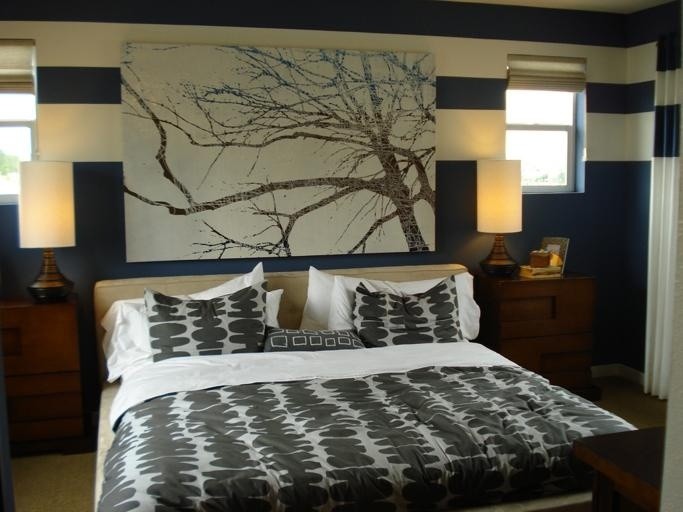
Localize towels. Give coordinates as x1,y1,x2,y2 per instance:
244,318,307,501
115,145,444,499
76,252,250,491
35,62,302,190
518,264,561,280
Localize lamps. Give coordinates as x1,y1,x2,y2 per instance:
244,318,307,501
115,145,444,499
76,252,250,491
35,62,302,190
475,157,522,277
18,158,78,302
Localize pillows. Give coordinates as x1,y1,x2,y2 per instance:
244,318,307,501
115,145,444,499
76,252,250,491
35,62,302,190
350,274,466,349
141,278,268,364
292,264,333,332
100,260,265,383
265,288,286,330
260,321,366,353
326,271,481,343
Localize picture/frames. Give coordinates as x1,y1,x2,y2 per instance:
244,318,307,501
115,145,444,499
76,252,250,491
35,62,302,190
537,236,569,276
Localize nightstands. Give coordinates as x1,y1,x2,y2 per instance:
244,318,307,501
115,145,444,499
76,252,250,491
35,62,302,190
2,296,99,456
471,266,598,394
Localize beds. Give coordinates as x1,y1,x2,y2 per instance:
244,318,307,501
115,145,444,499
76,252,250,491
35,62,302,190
86,251,638,512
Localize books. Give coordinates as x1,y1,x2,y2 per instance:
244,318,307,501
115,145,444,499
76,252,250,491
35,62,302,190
519,274,562,279
521,265,561,274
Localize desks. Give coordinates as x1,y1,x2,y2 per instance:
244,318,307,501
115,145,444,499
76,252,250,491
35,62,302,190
564,424,664,512
